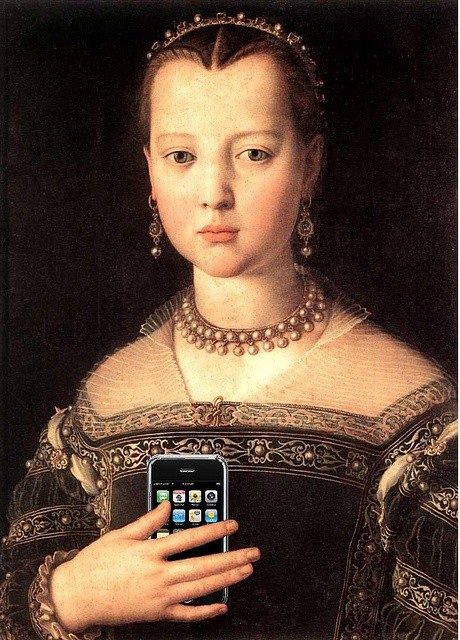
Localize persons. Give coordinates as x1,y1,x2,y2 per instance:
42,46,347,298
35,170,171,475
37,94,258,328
2,11,458,639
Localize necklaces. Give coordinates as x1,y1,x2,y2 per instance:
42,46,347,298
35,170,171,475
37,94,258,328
171,268,328,357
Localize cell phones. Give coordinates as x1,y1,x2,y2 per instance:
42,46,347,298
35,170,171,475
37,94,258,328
143,454,229,609
